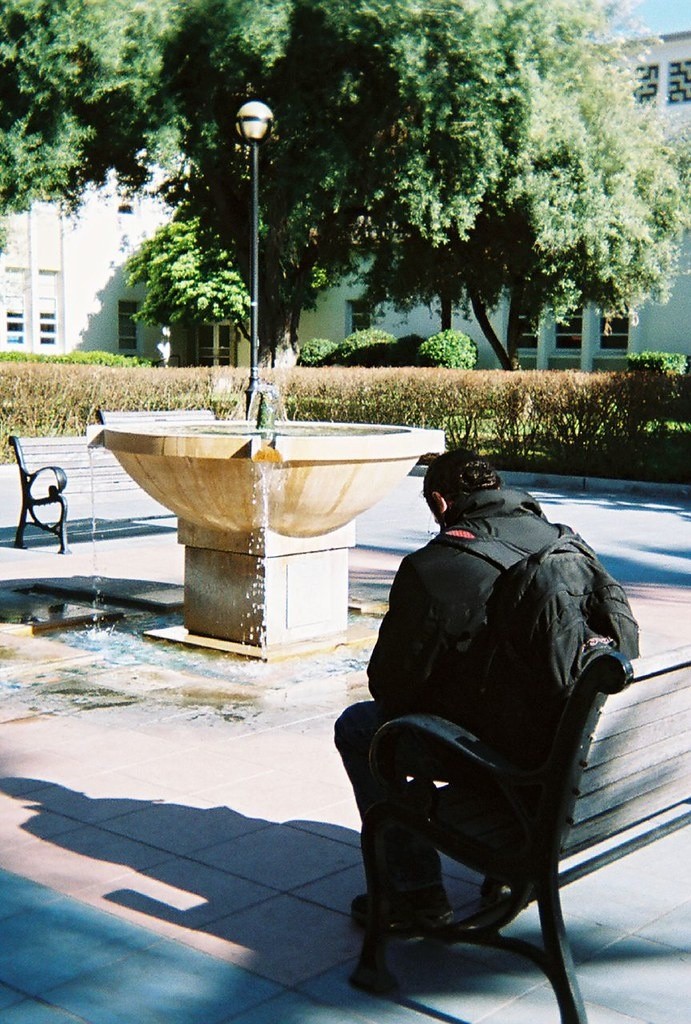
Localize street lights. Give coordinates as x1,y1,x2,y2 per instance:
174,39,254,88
237,100,275,419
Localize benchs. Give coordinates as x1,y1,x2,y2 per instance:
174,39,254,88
96,409,215,424
8,436,154,554
347,648,691,1024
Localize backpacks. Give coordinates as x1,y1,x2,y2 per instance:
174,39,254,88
429,524,640,764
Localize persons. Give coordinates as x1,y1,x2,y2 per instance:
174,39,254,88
331,447,596,933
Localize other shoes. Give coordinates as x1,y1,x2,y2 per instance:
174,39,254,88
350,886,453,932
